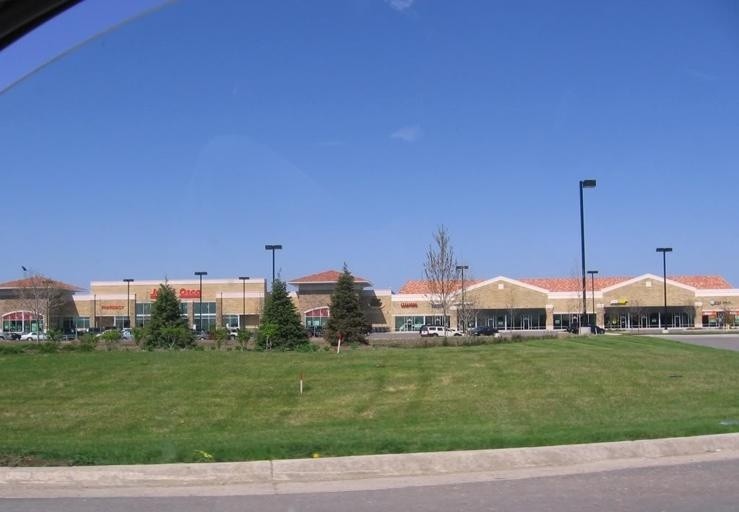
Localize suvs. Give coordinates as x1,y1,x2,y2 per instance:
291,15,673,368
565,322,606,335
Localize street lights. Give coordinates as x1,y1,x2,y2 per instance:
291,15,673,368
456,264,469,333
238,276,250,332
577,176,596,336
264,243,283,287
587,270,599,328
193,270,207,330
122,278,135,330
655,247,674,334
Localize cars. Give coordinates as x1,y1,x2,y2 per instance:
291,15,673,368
190,325,240,342
0,325,136,342
305,325,325,339
419,323,500,338
